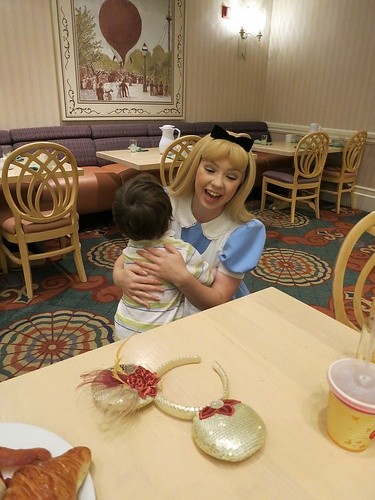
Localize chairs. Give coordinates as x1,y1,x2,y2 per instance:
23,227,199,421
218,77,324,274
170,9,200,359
160,135,201,186
0,141,86,297
333,210,375,331
320,130,368,214
260,131,329,222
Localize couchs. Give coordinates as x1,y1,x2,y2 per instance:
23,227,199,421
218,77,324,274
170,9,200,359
0,120,295,220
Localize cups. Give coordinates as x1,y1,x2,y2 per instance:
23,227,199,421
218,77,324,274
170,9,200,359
326,359,375,452
261,135,267,144
357,320,375,364
129,140,137,152
286,135,291,142
2,146,11,158
0,158,7,170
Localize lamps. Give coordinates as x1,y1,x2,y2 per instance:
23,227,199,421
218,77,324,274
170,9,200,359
240,26,264,43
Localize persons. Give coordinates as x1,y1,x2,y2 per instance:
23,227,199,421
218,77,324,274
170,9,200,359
112,124,267,310
113,172,214,340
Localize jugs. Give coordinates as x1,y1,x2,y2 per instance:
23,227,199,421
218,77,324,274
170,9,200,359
159,125,181,154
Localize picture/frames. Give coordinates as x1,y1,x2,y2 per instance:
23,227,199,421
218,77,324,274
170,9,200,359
50,0,187,121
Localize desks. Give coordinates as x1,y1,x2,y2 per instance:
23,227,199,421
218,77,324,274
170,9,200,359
0,286,375,500
95,144,258,171
253,141,342,156
0,155,84,184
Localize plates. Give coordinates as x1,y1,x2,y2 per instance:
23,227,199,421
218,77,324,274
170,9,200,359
0,423,96,500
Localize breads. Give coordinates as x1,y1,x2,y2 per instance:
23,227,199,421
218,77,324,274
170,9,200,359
0,445,92,500
